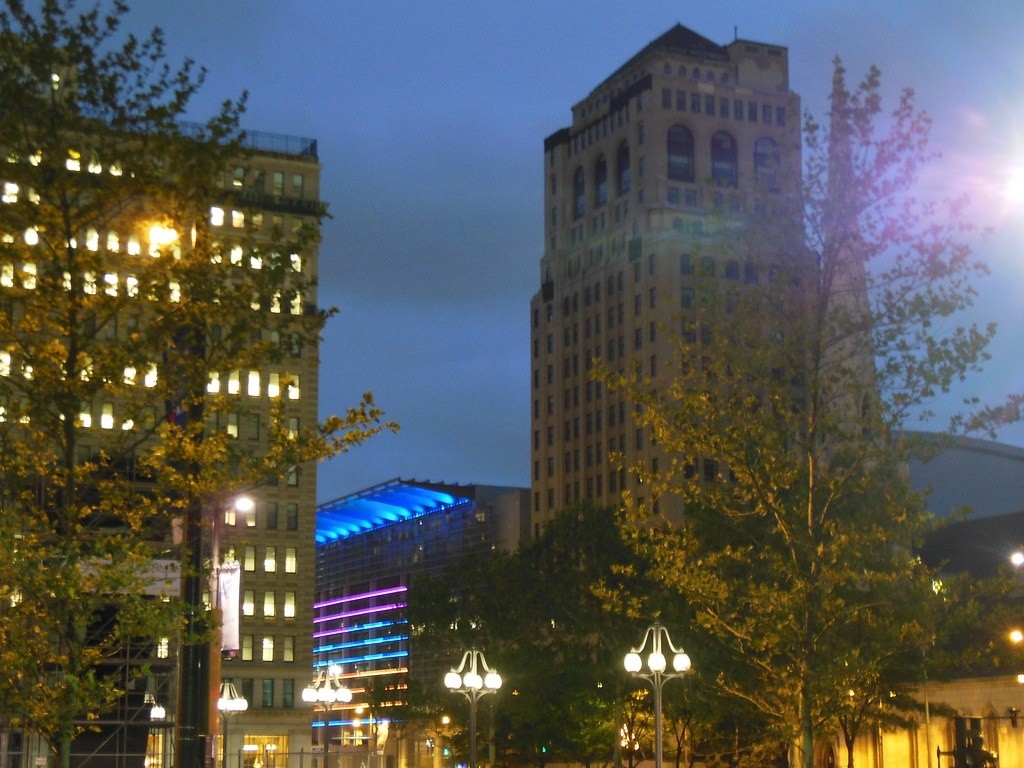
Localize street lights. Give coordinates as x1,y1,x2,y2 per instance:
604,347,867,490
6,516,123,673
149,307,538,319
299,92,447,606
623,606,694,768
219,679,248,768
301,667,352,768
444,641,503,768
151,702,165,768
206,496,255,768
